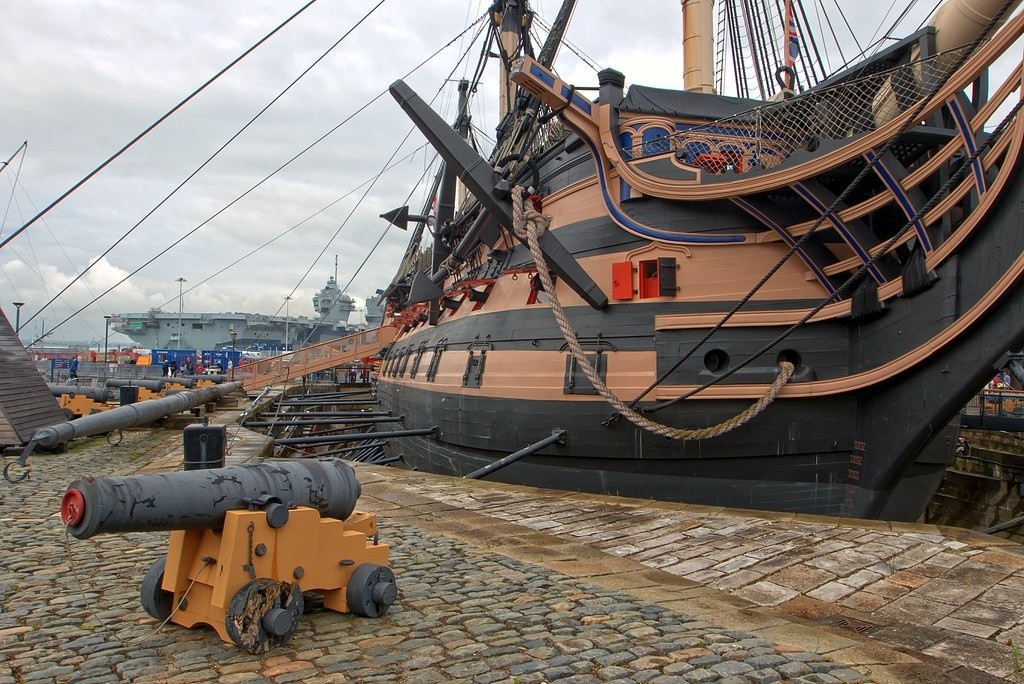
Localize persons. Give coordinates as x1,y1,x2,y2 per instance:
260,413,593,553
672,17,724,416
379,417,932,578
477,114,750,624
992,372,1010,390
68,353,80,385
361,369,366,383
227,358,232,369
161,357,223,378
350,364,357,383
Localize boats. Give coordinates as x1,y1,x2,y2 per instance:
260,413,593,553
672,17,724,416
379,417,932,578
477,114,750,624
110,255,387,357
368,0,1023,533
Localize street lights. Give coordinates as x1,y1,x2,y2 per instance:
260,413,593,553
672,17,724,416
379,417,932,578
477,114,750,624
229,329,237,381
284,296,294,349
102,314,111,379
176,277,186,348
12,302,25,336
